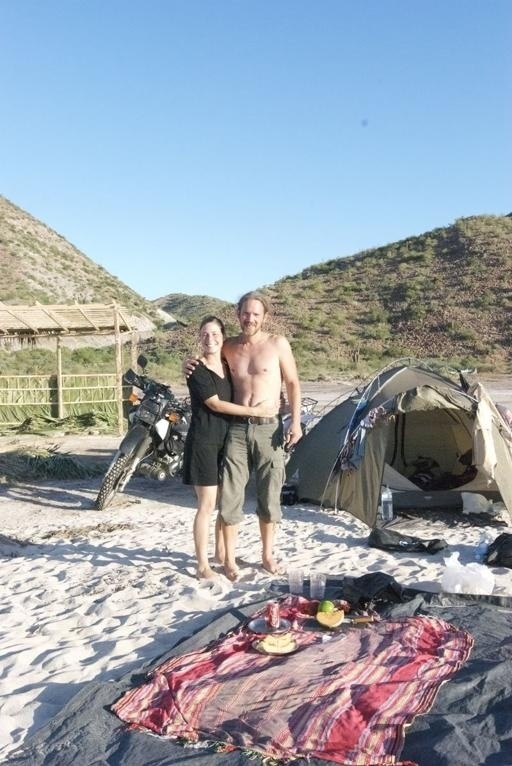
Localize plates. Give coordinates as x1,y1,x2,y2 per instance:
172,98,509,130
247,618,292,635
250,639,300,656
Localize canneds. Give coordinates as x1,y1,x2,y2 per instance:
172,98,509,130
266,601,280,629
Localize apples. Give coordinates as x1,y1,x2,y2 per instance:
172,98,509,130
318,601,334,612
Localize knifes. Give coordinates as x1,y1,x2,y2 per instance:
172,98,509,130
342,614,375,625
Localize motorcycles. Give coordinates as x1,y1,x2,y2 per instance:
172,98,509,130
95,349,318,511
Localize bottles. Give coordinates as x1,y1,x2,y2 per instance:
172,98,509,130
380,484,394,522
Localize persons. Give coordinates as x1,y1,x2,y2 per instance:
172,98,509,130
181,290,303,583
181,316,283,582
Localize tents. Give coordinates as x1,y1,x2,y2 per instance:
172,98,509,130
283,357,512,535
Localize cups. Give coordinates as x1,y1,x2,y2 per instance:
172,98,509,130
287,569,304,594
310,573,326,600
